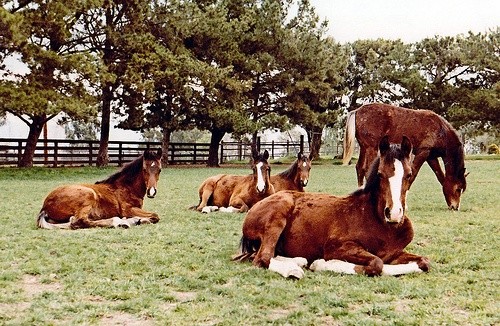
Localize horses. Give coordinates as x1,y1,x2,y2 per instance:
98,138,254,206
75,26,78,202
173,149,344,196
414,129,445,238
37,147,163,229
188,150,275,213
342,103,470,211
270,148,314,193
232,134,432,280
